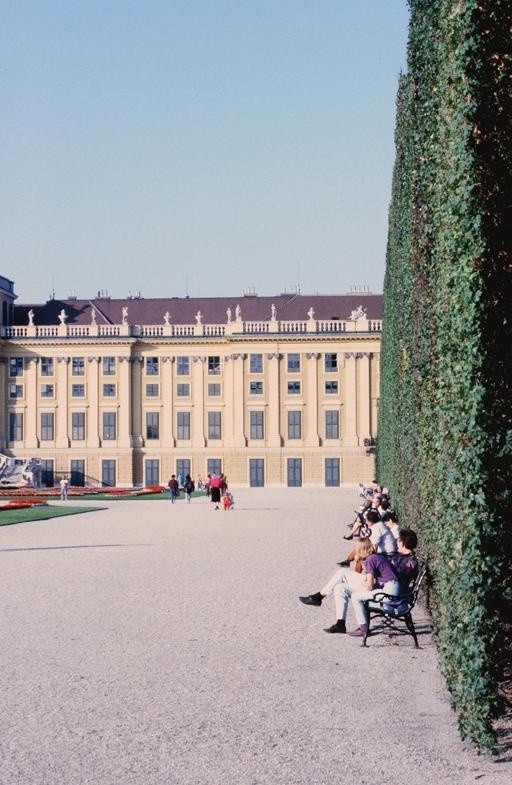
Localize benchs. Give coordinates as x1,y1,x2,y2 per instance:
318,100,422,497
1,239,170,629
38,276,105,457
360,556,428,650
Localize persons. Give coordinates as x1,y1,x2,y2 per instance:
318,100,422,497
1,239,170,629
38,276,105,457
333,477,403,570
58,474,70,501
298,527,420,634
346,537,403,637
166,470,233,513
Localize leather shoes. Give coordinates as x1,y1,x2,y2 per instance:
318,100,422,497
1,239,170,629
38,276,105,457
343,534,359,540
338,560,349,566
299,596,321,605
323,624,346,633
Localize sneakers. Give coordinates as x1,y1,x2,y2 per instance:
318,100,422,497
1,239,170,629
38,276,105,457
348,628,367,636
